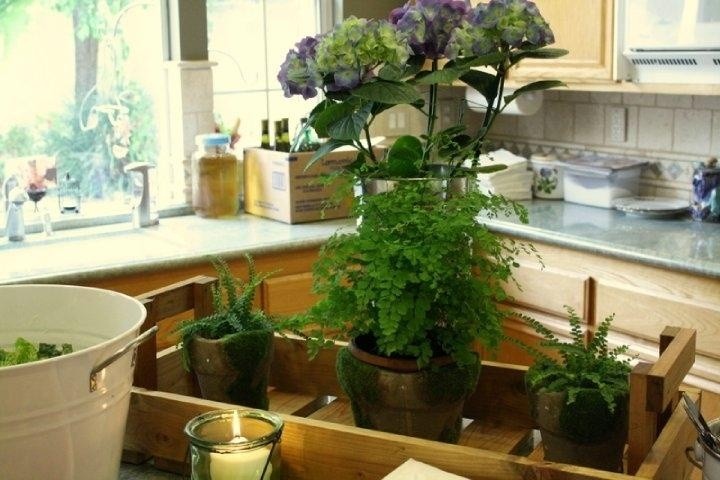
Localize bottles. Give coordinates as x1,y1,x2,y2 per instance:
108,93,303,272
260,118,309,152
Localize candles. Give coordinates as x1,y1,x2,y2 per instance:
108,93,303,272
208,411,272,480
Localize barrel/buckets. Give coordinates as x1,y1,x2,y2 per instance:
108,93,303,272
1,284,158,479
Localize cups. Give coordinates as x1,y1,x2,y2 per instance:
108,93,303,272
184,407,284,480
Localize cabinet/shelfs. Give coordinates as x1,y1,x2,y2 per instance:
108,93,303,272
419,0,616,85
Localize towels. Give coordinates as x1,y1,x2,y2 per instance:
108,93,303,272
468,148,534,201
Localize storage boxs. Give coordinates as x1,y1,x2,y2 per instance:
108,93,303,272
555,158,650,210
243,146,356,224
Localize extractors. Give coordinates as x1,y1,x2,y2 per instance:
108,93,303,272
618,44,720,86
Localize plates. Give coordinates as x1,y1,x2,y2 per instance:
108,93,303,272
610,198,690,217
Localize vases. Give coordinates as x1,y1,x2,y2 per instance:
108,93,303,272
353,165,469,237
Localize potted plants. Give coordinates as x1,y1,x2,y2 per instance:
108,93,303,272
272,131,546,445
156,252,314,406
487,305,641,473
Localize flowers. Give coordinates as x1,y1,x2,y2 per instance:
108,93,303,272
276,1,569,181
